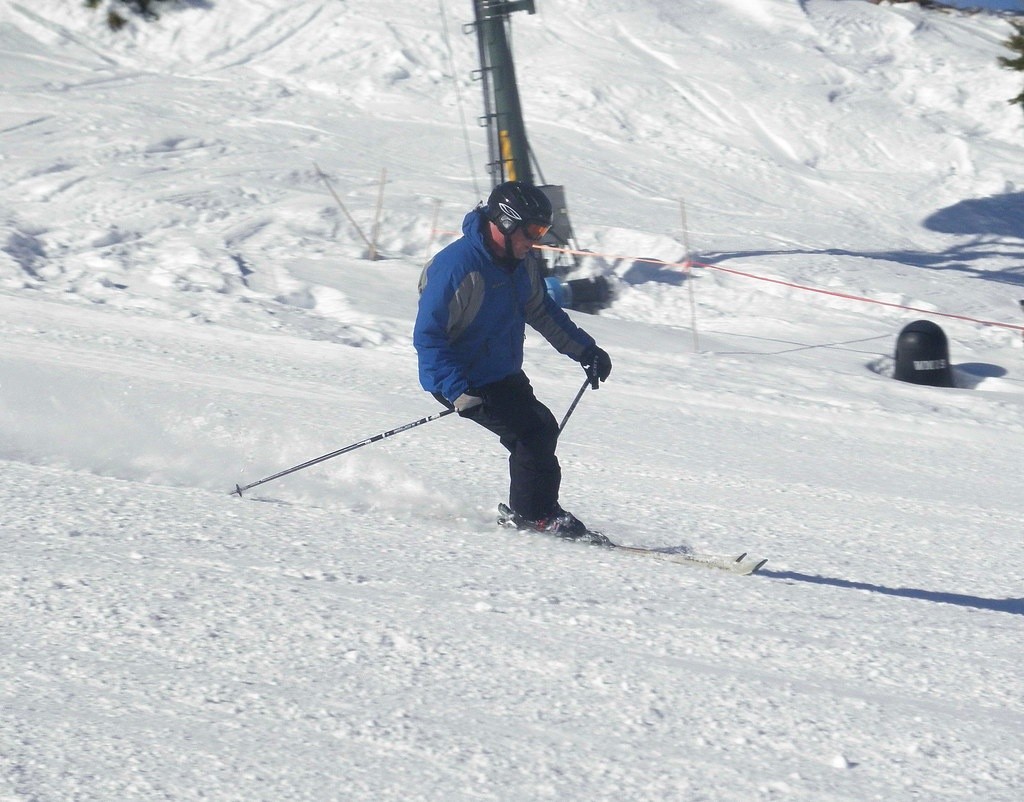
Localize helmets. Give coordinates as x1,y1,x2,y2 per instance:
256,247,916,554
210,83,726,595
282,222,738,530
487,181,552,237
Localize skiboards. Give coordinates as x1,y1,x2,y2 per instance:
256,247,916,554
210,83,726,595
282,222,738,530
499,499,768,578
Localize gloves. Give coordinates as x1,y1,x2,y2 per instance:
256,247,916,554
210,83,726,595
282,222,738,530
453,387,489,418
581,344,612,390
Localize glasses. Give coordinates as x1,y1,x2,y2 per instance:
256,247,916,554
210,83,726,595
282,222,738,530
518,218,552,242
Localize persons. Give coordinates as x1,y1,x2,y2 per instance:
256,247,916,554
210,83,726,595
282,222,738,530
413,182,614,538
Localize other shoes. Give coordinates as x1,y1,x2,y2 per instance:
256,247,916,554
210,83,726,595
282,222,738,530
511,506,587,542
